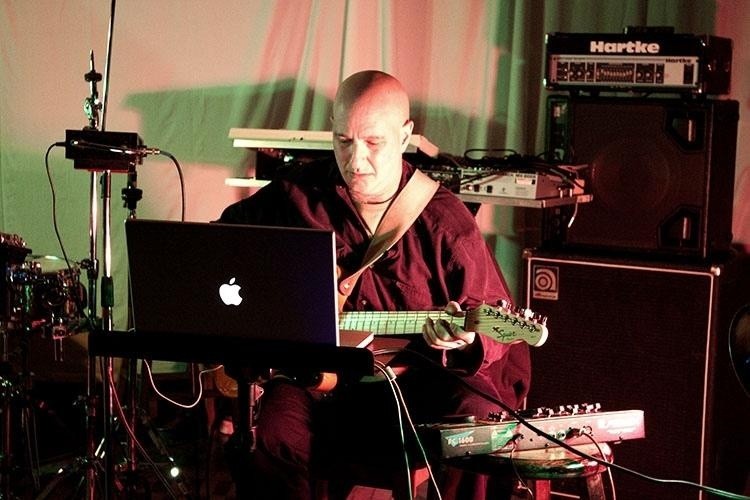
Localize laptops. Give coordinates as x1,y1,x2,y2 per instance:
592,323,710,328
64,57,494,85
124,219,374,348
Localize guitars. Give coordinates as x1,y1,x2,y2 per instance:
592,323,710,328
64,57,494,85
339,299,550,348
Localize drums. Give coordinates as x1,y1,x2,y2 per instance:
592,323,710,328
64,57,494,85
9,254,84,340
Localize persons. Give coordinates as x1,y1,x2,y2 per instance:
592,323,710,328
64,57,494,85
206,70,533,500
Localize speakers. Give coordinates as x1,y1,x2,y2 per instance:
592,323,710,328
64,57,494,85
518,245,750,500
545,93,741,264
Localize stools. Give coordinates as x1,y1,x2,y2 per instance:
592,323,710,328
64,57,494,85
455,442,614,500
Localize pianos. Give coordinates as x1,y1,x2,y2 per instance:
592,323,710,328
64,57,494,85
254,146,586,201
